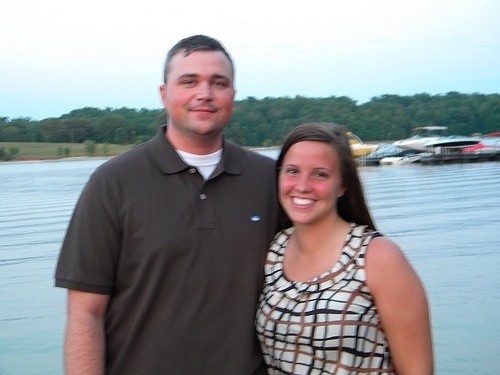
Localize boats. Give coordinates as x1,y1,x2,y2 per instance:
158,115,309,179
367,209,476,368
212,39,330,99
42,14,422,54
395,125,481,151
345,132,379,157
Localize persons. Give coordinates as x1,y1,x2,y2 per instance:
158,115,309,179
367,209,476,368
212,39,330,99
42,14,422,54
54,33,277,374
254,122,435,374
